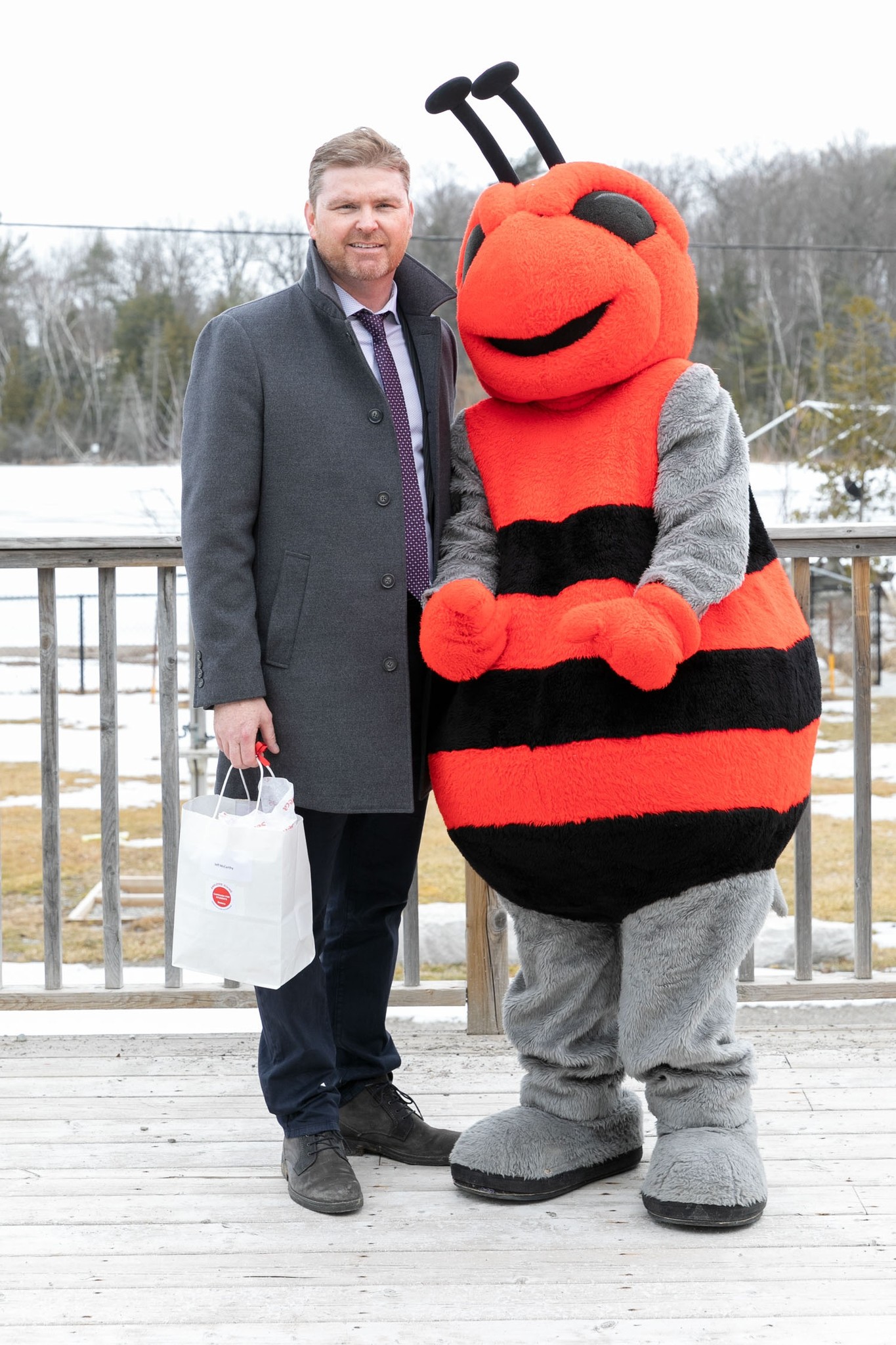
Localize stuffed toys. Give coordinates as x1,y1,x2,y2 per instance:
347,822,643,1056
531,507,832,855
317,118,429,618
423,62,820,1233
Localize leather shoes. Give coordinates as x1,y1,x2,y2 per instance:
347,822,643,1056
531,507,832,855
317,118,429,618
280,1127,364,1213
337,1083,462,1167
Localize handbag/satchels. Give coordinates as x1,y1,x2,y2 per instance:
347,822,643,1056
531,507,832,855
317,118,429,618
173,752,316,990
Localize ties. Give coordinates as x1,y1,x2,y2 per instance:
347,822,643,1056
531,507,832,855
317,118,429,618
351,308,430,603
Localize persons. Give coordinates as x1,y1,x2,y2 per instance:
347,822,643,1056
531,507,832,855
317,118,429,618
182,128,459,1213
